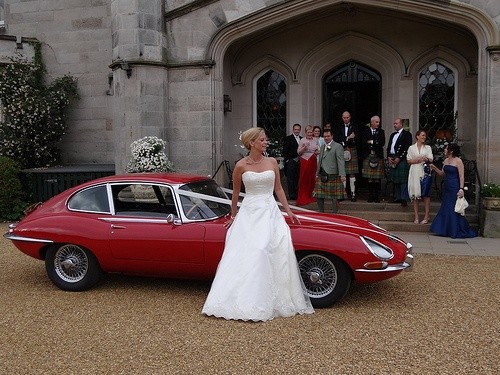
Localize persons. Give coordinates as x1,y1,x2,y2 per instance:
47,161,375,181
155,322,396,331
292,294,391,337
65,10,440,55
201,127,316,322
386,118,412,207
406,129,435,224
430,144,477,239
296,125,320,206
314,129,347,214
337,111,359,202
312,123,331,162
282,124,303,201
360,116,386,203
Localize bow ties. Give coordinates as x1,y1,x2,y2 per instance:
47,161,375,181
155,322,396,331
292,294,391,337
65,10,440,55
394,132,399,134
346,125,349,127
324,145,331,152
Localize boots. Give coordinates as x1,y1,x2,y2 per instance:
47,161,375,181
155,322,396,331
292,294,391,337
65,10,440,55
350,177,357,202
375,181,381,203
367,182,374,202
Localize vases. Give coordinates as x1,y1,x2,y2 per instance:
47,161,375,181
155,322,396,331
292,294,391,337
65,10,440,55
129,184,168,202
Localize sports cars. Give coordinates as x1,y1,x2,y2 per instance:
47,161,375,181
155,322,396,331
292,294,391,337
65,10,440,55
2,172,414,309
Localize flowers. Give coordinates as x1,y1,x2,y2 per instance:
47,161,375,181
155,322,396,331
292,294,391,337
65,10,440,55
124,136,175,174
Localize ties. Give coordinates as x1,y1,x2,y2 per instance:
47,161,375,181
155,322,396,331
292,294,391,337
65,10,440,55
373,131,375,137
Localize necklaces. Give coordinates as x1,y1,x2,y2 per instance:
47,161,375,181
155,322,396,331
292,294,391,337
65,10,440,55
249,156,264,165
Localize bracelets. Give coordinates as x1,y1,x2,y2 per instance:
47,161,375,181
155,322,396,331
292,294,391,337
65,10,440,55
231,216,234,220
459,187,464,190
287,210,292,215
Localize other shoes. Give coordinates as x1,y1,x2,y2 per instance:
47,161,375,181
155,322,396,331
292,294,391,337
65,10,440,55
401,199,407,206
414,216,419,224
390,199,402,203
420,216,430,224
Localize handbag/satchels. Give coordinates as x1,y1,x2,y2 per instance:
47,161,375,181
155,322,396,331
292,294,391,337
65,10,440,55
455,196,469,216
321,172,328,182
343,151,351,161
369,157,379,168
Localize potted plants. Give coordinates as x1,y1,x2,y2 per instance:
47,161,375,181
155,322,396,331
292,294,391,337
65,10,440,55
480,183,500,210
